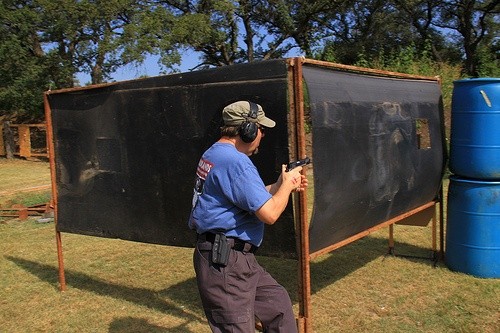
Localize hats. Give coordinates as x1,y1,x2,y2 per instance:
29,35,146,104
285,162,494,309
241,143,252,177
221,100,276,127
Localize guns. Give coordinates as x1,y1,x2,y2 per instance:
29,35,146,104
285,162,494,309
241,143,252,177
288,156,310,172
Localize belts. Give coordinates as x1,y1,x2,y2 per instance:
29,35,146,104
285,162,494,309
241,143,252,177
198,231,257,253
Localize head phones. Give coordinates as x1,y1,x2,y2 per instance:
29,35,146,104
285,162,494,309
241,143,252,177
239,99,258,144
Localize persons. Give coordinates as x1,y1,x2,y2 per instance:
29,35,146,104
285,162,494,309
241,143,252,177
188,100,309,333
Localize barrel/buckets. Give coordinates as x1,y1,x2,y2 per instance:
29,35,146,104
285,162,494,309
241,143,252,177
445,179,500,279
448,78,500,179
